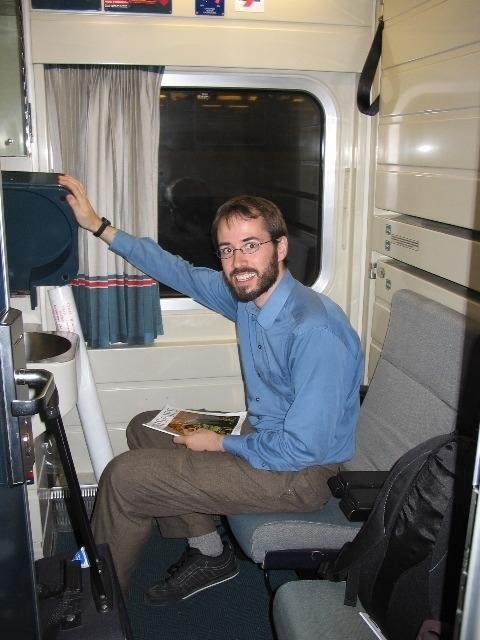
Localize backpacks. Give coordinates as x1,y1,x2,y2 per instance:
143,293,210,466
327,426,459,640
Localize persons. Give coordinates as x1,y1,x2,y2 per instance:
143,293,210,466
54,172,365,606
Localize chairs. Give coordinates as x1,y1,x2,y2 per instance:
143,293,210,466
224,288,467,579
260,489,465,639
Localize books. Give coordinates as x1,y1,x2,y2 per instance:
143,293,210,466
142,403,249,438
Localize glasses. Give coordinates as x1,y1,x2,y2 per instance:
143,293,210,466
215,238,275,261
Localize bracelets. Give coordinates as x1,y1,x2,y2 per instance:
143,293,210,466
92,216,112,238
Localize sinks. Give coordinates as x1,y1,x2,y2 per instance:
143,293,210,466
23,330,79,439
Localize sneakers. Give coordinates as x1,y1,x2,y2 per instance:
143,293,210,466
141,538,242,609
